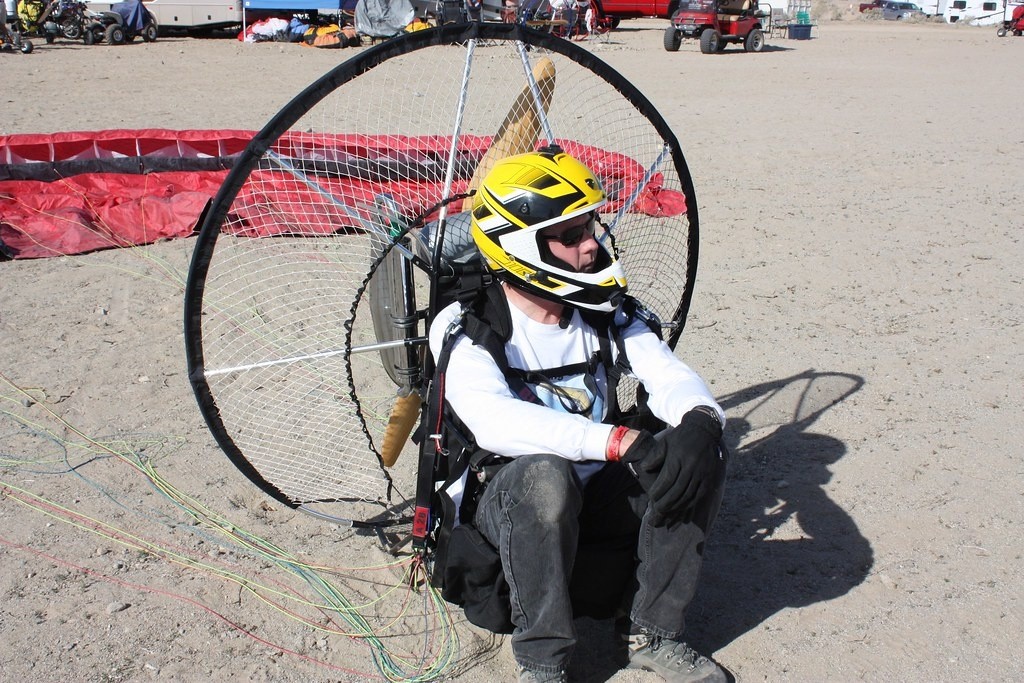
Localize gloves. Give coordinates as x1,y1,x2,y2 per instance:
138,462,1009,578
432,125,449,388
642,409,729,533
619,427,665,496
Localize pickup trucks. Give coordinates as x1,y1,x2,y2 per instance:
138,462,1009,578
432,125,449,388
859,0,926,23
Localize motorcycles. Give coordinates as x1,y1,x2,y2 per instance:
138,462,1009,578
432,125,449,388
664,0,772,54
44,1,157,46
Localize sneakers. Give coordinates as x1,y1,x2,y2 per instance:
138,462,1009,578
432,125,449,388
610,615,729,683
514,660,568,683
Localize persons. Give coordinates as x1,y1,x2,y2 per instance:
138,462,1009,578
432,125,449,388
466,0,481,22
425,145,731,683
0,0,7,26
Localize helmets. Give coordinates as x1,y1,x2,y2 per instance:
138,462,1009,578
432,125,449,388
469,143,629,312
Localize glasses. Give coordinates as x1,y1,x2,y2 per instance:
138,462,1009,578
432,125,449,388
543,209,596,246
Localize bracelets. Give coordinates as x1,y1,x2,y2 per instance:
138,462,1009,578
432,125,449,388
607,425,630,461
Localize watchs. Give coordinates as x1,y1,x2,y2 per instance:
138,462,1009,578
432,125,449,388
695,406,723,426
